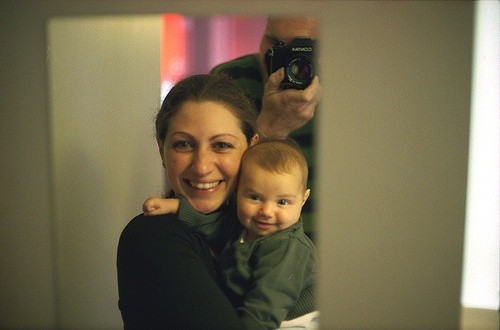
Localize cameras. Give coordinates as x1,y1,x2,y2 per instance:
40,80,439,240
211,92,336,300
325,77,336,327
265,37,317,89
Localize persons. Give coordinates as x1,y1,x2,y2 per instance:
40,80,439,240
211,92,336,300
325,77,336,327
208,13,322,245
116,75,260,330
143,136,321,330
